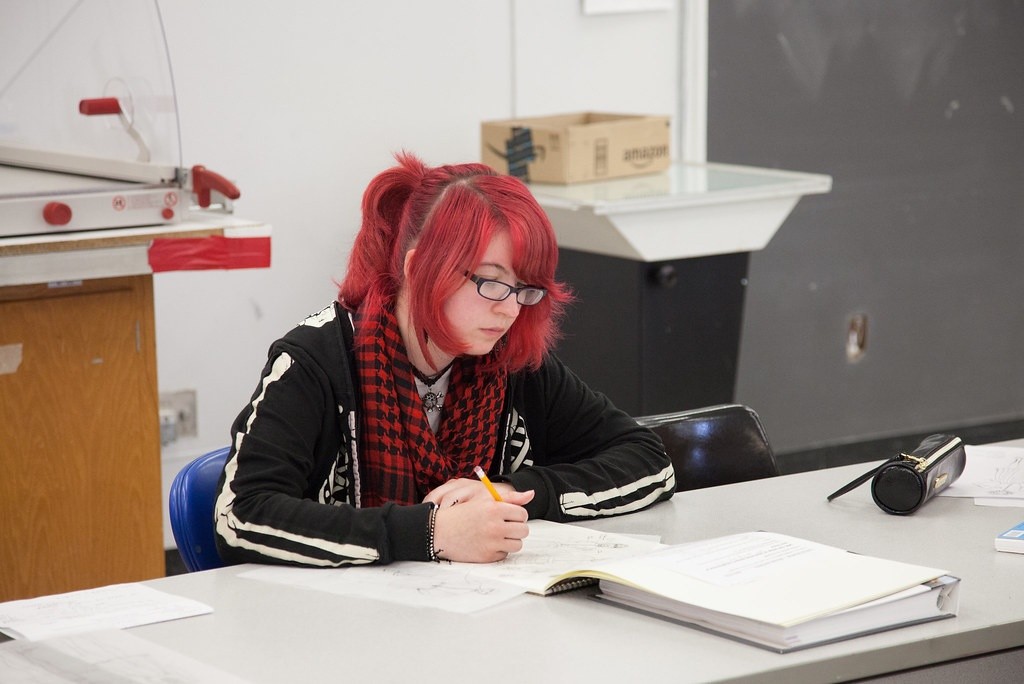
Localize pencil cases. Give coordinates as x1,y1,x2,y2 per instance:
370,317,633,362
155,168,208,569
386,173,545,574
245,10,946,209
827,432,968,517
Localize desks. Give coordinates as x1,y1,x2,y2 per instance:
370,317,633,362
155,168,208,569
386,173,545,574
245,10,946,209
0,437,1024,684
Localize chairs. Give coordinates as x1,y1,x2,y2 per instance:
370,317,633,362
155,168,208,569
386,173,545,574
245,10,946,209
632,403,780,493
169,446,234,569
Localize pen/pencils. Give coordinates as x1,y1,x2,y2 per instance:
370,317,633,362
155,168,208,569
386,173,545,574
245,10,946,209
473,466,504,502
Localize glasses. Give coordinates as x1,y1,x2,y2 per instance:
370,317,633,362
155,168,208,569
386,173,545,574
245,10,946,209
455,264,546,306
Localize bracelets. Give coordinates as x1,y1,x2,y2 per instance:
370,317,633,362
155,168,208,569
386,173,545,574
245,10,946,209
426,504,440,563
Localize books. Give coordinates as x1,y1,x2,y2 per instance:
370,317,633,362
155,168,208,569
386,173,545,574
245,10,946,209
527,521,1024,656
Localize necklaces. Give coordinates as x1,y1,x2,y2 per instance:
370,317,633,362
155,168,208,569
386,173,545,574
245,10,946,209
407,360,452,414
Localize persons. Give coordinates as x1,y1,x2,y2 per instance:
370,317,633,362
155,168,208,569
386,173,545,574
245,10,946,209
213,150,675,574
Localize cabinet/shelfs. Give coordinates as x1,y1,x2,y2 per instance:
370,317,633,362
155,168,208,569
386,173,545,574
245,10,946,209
0,276,165,603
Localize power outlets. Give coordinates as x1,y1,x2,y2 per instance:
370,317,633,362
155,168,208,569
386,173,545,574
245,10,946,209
158,385,199,441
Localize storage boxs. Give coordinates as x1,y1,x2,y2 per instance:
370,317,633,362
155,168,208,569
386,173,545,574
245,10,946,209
482,110,670,184
